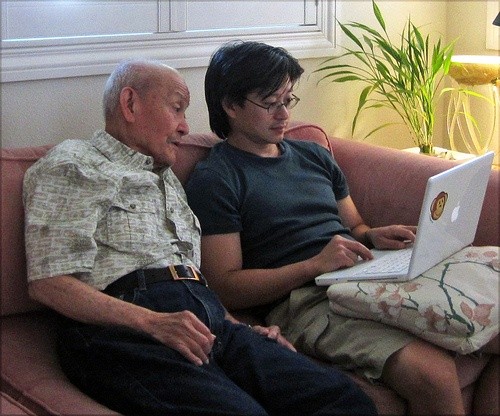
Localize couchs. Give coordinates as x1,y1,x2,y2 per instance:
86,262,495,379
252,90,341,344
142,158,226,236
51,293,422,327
0,121,500,416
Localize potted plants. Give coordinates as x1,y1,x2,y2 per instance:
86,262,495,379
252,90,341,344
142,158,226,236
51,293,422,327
306,0,478,162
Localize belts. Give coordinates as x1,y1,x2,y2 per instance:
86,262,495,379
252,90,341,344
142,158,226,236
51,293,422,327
100,264,207,297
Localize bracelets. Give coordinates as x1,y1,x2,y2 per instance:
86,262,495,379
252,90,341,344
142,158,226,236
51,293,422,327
239,322,251,329
364,230,373,248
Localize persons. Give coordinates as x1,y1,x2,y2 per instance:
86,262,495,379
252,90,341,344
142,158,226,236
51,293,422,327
183,40,500,416
22,55,380,416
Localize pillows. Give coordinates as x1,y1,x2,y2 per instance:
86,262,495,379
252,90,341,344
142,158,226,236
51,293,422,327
327,246,500,355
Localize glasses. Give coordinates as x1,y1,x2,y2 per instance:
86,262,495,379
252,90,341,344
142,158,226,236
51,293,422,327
240,94,300,114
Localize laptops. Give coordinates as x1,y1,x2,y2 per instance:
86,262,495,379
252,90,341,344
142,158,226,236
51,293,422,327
315,151,494,286
